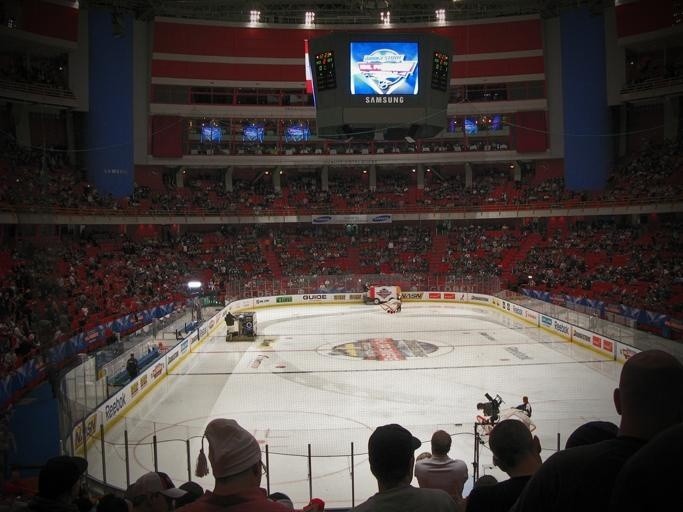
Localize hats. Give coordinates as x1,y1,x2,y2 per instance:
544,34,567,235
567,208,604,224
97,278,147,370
368,424,421,465
205,419,260,480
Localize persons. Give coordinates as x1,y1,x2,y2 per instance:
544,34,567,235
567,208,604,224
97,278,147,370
510,395,531,417
534,216,681,339
0,136,198,398
534,165,681,208
0,402,204,512
510,349,681,510
465,474,498,504
199,219,532,292
414,429,469,504
200,171,532,216
476,417,543,511
353,423,456,510
180,417,293,510
